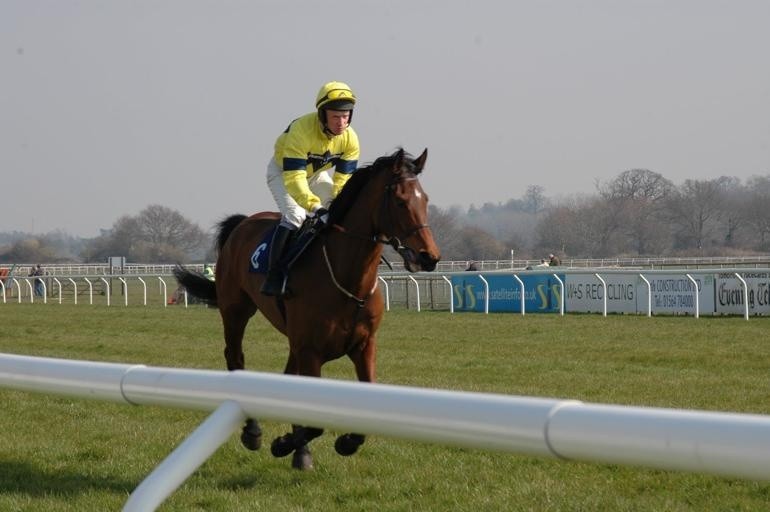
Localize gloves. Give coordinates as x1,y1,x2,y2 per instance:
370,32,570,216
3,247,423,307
312,204,328,223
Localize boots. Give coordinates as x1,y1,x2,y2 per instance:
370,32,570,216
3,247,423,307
260,227,292,295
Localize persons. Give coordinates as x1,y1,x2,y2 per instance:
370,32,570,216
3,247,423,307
548,253,560,266
202,264,213,280
536,257,549,268
28,267,43,297
258,79,361,300
466,260,478,272
37,265,43,292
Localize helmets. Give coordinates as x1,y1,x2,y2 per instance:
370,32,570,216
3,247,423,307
315,83,356,125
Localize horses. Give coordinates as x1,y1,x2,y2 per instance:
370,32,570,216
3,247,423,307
171,146,442,472
